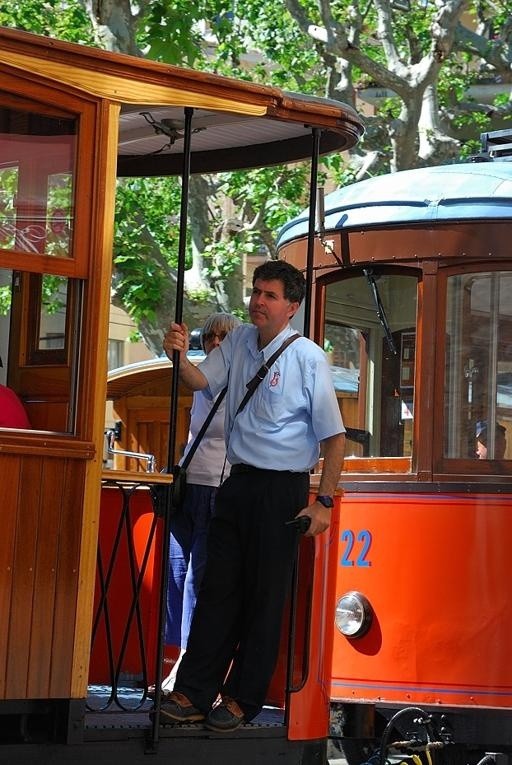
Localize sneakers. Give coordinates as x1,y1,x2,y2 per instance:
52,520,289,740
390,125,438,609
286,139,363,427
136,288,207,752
148,690,244,733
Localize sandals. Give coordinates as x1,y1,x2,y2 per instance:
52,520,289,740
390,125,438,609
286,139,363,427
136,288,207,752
148,683,169,701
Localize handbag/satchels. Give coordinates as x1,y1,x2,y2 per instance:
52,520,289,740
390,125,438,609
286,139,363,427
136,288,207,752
148,464,187,518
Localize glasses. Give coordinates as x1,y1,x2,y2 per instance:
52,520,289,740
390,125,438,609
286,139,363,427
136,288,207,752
201,333,226,341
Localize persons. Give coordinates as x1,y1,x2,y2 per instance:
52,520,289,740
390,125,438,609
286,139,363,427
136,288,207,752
476,420,506,458
